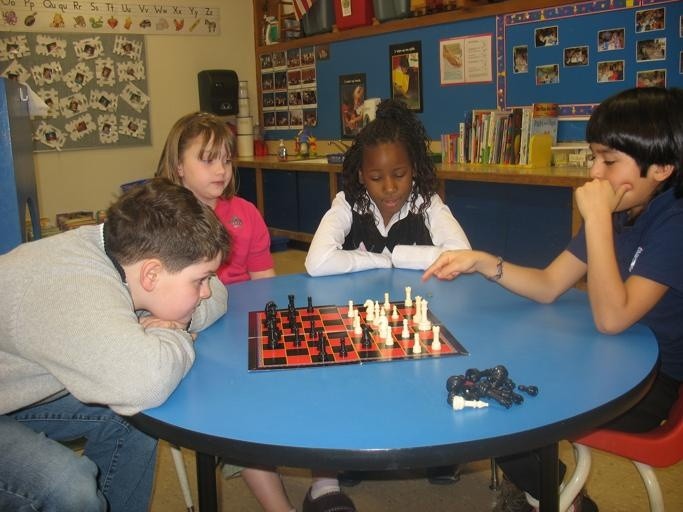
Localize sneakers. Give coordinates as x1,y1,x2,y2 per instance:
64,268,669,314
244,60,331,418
428,464,460,483
303,486,355,512
493,491,598,512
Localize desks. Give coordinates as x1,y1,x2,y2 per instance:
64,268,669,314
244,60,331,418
122,260,662,512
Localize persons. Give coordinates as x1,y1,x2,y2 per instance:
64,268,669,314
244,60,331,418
422,84,683,512
393,55,411,99
304,100,477,511
156,111,301,512
1,173,234,512
343,83,365,128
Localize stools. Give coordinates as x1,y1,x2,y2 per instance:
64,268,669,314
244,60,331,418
526,376,682,512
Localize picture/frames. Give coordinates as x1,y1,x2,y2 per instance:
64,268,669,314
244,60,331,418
388,40,423,113
338,72,366,138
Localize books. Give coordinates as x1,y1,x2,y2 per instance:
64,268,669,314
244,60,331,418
440,102,559,167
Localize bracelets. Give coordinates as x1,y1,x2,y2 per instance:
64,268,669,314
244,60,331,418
488,252,507,286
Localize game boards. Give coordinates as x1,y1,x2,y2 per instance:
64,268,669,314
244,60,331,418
248,298,471,371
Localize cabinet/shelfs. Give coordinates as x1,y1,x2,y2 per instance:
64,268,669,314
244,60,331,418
260,168,330,235
